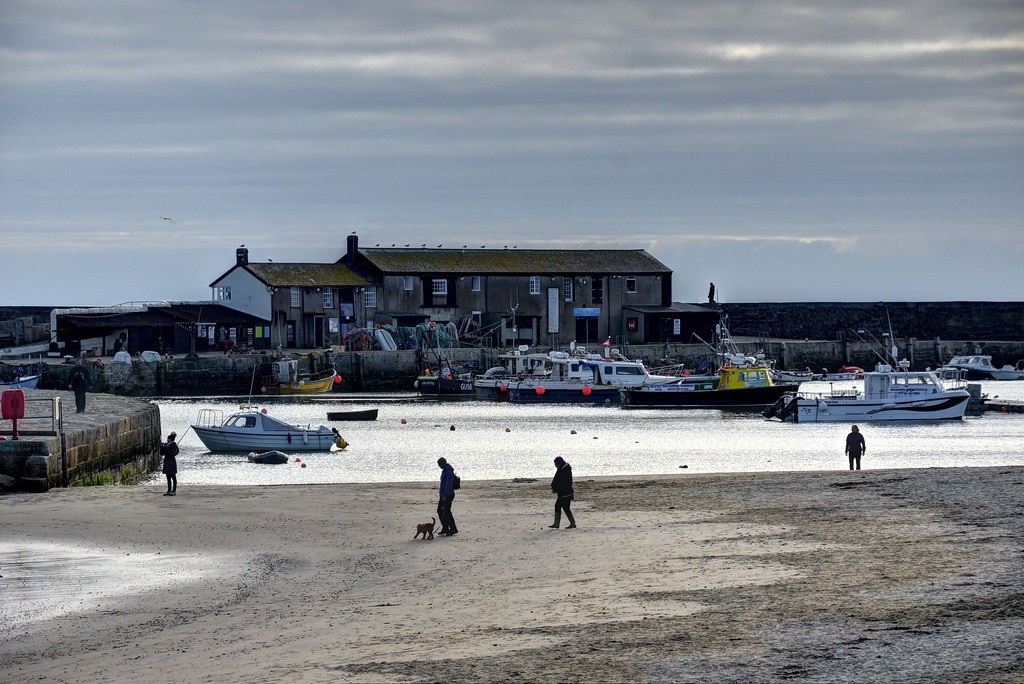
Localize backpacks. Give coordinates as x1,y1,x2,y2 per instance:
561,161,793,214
453,471,462,490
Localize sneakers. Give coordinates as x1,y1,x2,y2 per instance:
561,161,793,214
565,523,576,529
438,526,450,535
446,525,458,536
162,490,176,496
548,522,559,528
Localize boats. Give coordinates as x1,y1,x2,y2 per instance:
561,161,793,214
191,366,337,454
761,310,971,423
262,307,337,393
0,365,39,389
932,336,1024,383
405,270,907,408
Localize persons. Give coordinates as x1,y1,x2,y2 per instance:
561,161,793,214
68,359,93,414
548,457,577,529
845,425,866,470
436,457,461,537
159,432,179,496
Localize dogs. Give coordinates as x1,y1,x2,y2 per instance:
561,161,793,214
413,516,436,540
